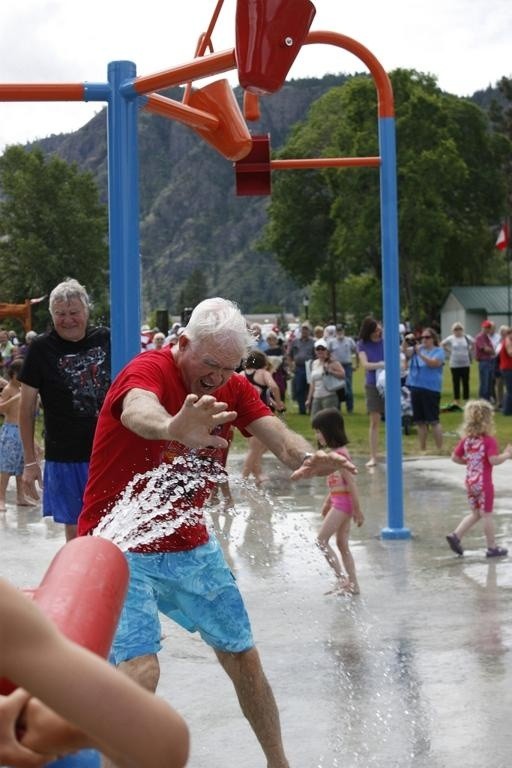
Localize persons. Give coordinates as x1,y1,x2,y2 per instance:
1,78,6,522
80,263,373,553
0,330,43,418
247,322,360,413
357,317,386,466
0,572,192,765
141,323,186,353
400,332,421,386
441,322,475,404
75,296,357,768
444,398,512,557
305,408,366,599
305,340,345,450
402,329,446,453
476,320,512,414
240,350,283,486
0,359,37,513
18,281,113,547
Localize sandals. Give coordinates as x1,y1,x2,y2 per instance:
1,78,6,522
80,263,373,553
444,531,509,558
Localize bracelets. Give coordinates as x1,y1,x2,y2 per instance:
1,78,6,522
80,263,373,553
302,453,311,460
26,462,37,466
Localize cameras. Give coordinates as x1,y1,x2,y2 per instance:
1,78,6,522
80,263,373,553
405,338,420,346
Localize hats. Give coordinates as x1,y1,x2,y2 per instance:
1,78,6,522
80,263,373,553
480,318,493,328
299,319,347,351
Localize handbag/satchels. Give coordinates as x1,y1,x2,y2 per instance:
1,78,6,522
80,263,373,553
374,364,394,398
400,387,414,418
322,371,346,393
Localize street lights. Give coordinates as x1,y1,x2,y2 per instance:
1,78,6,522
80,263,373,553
301,293,311,321
